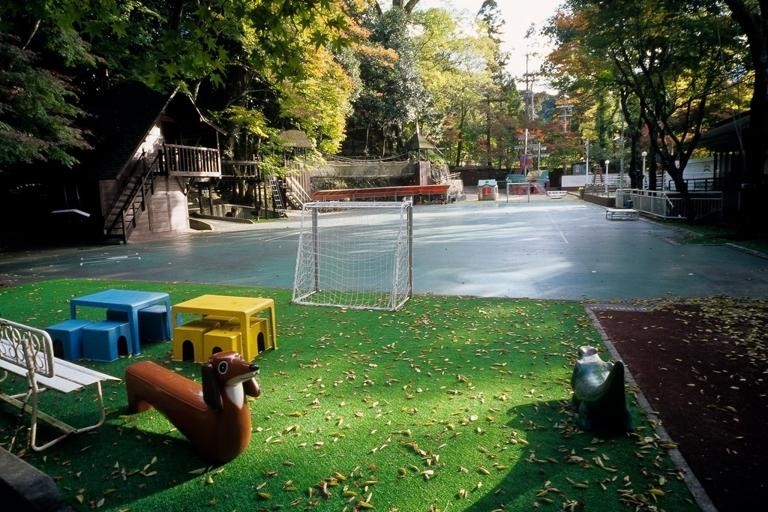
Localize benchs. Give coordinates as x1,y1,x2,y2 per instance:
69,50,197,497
0,313,121,453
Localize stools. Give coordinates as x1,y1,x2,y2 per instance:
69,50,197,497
46,304,271,364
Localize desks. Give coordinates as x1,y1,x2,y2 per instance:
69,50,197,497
71,288,277,361
605,209,639,221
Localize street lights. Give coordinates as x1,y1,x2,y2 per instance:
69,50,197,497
532,79,540,120
642,151,647,190
560,95,569,132
605,160,609,193
526,51,539,115
